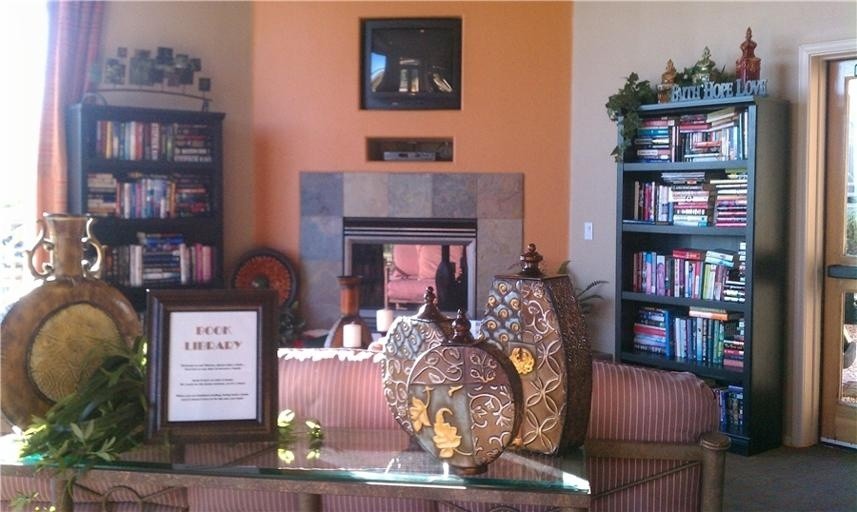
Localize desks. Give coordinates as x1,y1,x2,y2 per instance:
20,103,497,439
4,425,733,511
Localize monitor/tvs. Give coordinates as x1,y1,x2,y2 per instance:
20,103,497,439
360,17,463,109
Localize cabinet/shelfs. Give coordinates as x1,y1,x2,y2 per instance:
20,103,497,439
62,101,228,313
611,75,793,457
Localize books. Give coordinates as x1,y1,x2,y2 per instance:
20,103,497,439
712,382,742,429
88,116,216,285
628,106,751,370
